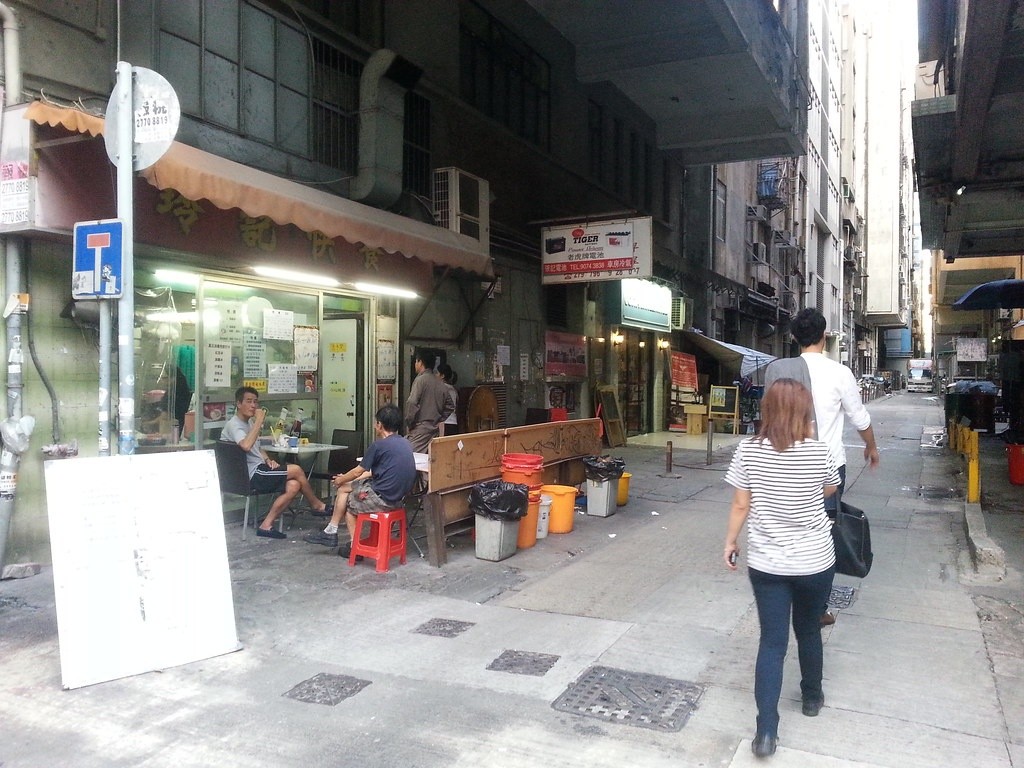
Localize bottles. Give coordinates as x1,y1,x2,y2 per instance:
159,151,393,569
274,407,288,435
289,407,304,438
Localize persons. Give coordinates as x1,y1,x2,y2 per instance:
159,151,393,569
434,364,459,436
220,386,335,539
403,350,454,510
302,405,417,561
142,364,190,436
790,308,878,627
723,377,841,757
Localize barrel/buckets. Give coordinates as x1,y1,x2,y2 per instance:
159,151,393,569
616,472,632,506
499,452,578,549
1006,445,1024,486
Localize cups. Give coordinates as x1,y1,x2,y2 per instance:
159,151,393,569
271,433,280,446
288,437,298,447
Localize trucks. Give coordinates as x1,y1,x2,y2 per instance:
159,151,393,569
906,359,934,393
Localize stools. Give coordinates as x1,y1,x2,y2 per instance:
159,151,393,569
349,508,407,572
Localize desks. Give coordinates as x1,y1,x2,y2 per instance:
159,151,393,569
356,453,430,558
263,443,348,532
466,384,499,432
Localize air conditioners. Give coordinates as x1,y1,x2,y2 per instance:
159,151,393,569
749,205,767,218
433,167,490,255
752,242,766,262
671,297,694,331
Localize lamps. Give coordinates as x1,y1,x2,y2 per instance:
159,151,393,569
660,341,669,351
614,334,624,346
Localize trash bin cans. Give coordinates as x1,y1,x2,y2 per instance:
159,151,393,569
582,454,625,517
467,481,528,562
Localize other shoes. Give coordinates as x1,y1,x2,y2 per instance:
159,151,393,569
312,503,335,515
752,731,776,756
803,690,824,716
257,526,287,538
819,612,835,628
303,530,339,547
338,542,363,561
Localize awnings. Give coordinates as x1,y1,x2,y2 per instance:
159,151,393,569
22,100,494,278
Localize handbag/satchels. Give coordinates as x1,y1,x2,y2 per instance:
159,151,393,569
825,491,874,579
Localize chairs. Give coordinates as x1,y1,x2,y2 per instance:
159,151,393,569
303,429,362,525
215,441,285,541
